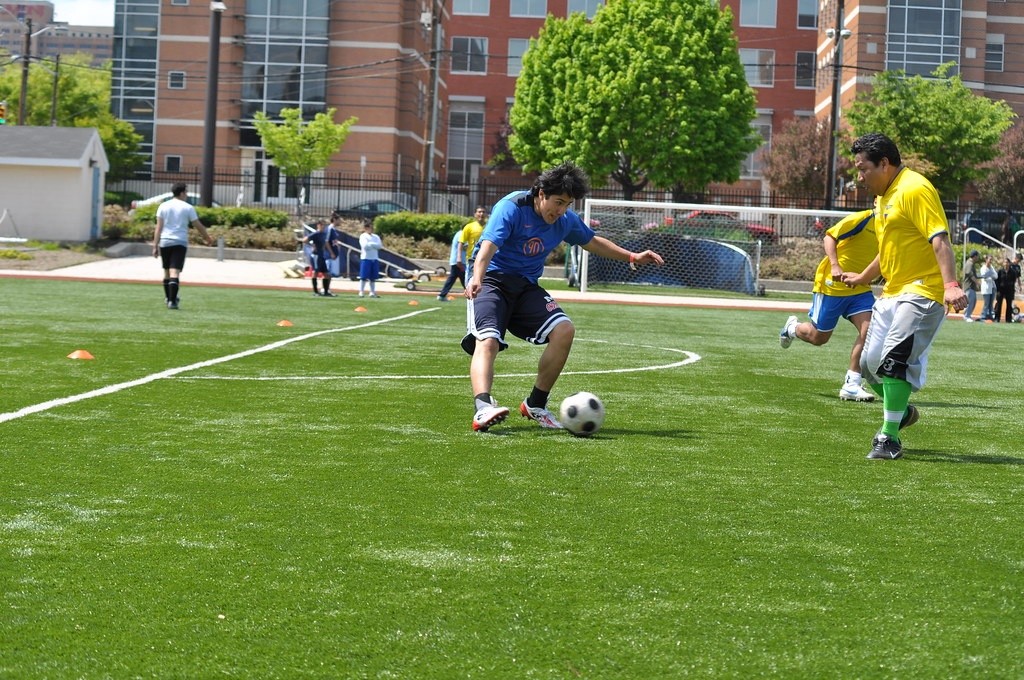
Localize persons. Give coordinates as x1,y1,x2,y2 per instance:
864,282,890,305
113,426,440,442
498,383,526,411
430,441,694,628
153,182,213,309
964,250,981,322
779,197,879,401
460,162,665,432
359,222,381,298
840,134,969,459
320,213,342,297
457,205,489,270
437,223,468,301
303,220,326,295
980,253,1024,324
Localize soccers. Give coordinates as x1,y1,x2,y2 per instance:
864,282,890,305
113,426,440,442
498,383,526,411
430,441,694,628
1014,315,1022,322
560,391,606,436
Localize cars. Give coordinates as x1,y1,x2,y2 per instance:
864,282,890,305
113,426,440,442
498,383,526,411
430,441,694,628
954,206,1015,249
332,198,414,222
128,191,224,220
663,211,780,247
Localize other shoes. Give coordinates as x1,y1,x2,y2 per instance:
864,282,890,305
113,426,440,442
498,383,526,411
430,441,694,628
325,292,336,297
314,293,321,297
964,315,973,323
167,301,177,308
165,297,180,304
359,293,364,297
369,293,380,298
437,296,447,301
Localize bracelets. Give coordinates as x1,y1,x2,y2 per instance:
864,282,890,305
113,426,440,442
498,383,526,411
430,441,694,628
630,253,636,270
944,281,962,289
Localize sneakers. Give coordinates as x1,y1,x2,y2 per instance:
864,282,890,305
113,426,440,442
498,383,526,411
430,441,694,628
866,436,902,461
473,396,509,432
871,405,919,447
839,383,874,402
780,316,798,349
520,397,565,429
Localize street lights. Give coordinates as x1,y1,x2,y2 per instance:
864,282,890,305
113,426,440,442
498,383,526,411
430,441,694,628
824,0,852,217
8,51,60,127
18,17,69,126
